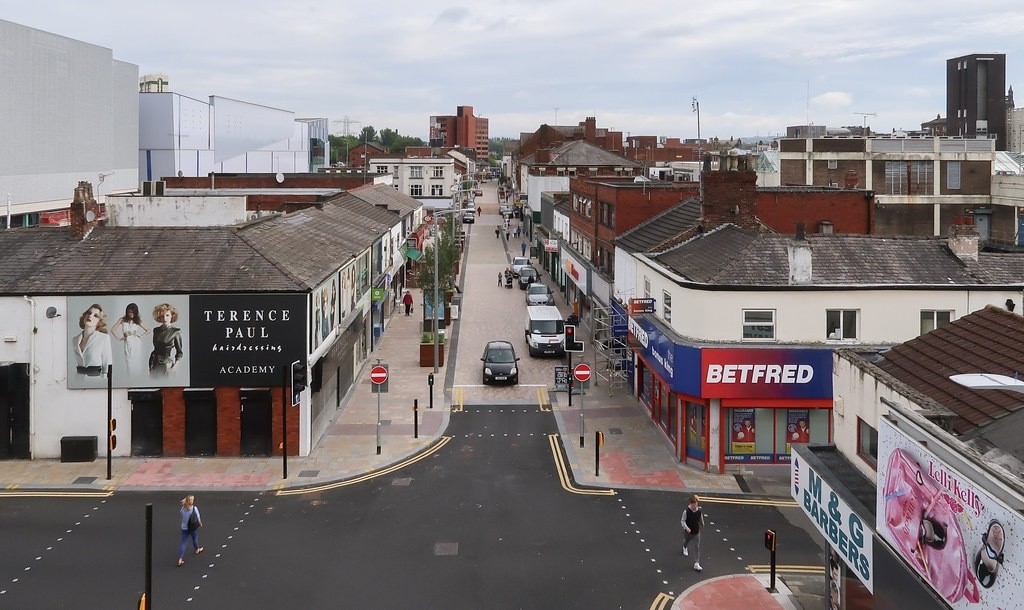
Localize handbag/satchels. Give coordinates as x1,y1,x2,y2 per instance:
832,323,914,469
188,505,200,531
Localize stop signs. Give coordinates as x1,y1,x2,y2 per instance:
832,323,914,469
574,364,591,381
370,366,388,384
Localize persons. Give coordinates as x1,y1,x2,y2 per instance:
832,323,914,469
177,494,204,567
110,303,151,376
72,304,112,379
513,227,517,239
794,419,809,443
569,312,579,327
505,228,510,241
494,225,502,239
403,291,413,316
680,494,705,571
148,302,184,380
504,267,514,281
517,226,521,237
741,419,755,442
477,207,481,216
520,240,526,256
528,275,535,284
497,272,502,287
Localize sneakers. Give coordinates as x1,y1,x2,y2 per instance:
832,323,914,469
683,546,689,555
693,563,702,570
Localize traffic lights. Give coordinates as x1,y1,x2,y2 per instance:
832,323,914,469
565,325,584,352
291,360,305,406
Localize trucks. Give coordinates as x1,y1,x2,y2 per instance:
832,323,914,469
523,306,565,357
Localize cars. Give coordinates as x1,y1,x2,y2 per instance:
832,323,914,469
526,283,555,306
503,208,513,217
518,266,542,288
499,206,508,215
510,257,534,277
475,189,482,196
480,340,520,385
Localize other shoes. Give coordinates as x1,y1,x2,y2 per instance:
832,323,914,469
195,547,203,554
177,560,184,566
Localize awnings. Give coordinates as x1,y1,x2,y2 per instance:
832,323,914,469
406,248,424,262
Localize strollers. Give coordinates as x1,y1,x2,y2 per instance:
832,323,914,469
505,276,513,289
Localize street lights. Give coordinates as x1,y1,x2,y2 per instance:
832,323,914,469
452,170,481,246
433,208,476,373
